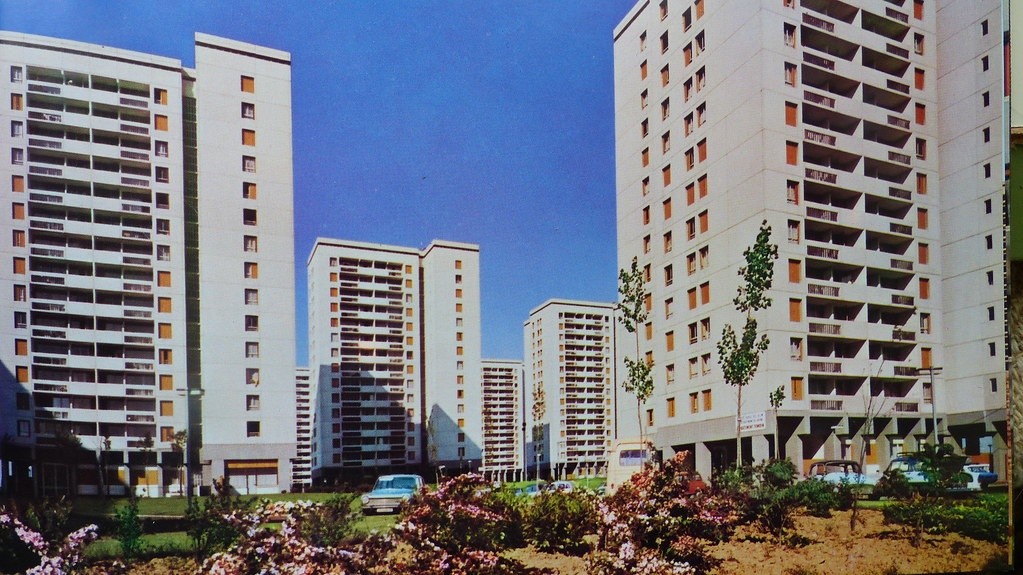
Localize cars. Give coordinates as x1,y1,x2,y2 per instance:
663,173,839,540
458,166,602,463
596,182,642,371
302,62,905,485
360,473,425,516
803,460,877,501
881,453,999,493
